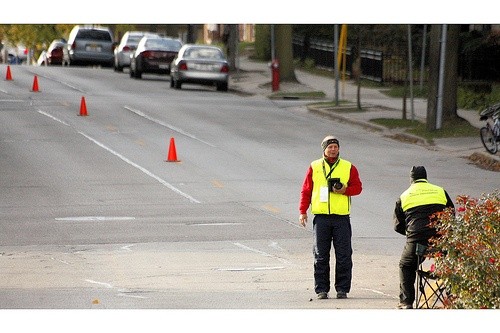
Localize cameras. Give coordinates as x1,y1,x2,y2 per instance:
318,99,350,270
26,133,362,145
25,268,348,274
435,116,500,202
327,178,342,192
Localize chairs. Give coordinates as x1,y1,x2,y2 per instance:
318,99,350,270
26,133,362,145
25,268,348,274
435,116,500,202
415,243,447,309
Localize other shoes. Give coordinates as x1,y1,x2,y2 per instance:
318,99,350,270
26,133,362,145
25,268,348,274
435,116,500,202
317,292,328,299
336,290,347,299
396,303,413,309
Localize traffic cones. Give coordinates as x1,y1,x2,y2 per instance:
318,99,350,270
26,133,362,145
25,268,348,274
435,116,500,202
166,137,181,162
77,96,90,116
5,65,14,81
29,74,42,93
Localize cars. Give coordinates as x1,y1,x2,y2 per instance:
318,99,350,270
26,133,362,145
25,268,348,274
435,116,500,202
170,44,229,90
37,40,66,66
62,24,114,67
130,36,183,79
114,31,160,72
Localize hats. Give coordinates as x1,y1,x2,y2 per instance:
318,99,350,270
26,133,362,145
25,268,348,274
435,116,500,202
321,135,340,152
409,165,427,180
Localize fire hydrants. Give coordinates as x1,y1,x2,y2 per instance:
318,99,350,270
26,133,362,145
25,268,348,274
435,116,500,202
268,60,279,91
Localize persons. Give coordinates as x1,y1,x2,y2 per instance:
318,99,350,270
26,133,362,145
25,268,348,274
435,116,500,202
393,166,455,309
299,135,362,298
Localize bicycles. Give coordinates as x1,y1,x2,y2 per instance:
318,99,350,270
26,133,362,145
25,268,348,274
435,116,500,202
479,104,500,154
